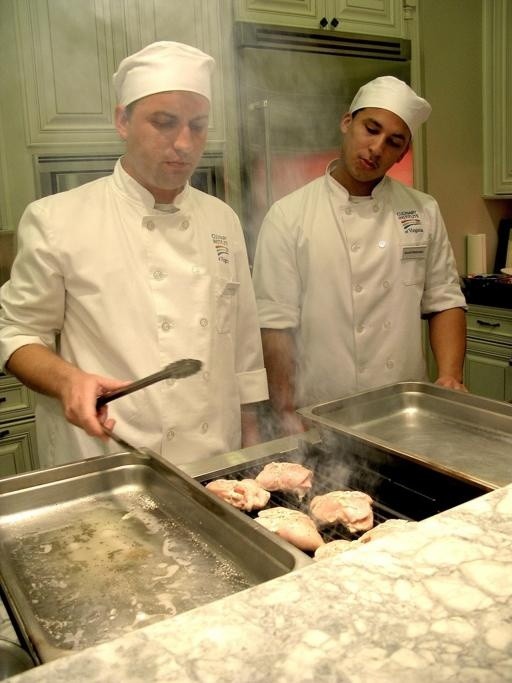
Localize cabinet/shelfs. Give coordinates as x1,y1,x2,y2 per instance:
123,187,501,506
461,305,512,405
234,0,407,39
0,378,42,476
12,0,232,155
479,0,511,202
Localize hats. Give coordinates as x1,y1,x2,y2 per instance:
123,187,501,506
348,75,432,141
112,40,217,109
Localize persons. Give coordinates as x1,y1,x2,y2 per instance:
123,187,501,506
1,39,273,468
250,76,471,436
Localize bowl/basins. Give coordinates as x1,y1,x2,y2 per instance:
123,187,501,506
459,273,507,287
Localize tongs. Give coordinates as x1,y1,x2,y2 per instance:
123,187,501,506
93,357,203,467
